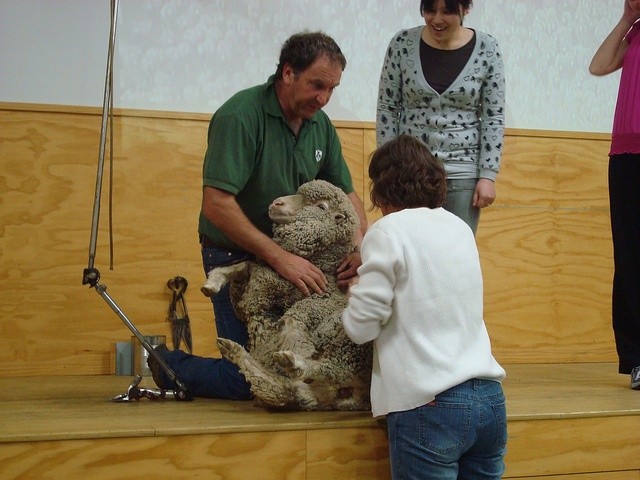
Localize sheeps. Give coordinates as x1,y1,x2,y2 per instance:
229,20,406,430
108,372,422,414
200,177,375,413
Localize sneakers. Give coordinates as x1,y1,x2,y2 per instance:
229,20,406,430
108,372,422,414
629,366,640,389
146,344,169,388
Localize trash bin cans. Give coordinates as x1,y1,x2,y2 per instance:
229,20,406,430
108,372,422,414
131,335,166,377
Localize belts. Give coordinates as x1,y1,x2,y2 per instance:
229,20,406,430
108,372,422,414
198,236,220,249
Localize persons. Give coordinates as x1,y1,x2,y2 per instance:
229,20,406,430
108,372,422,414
375,0,506,236
149,28,367,400
589,1,640,389
342,136,506,479
